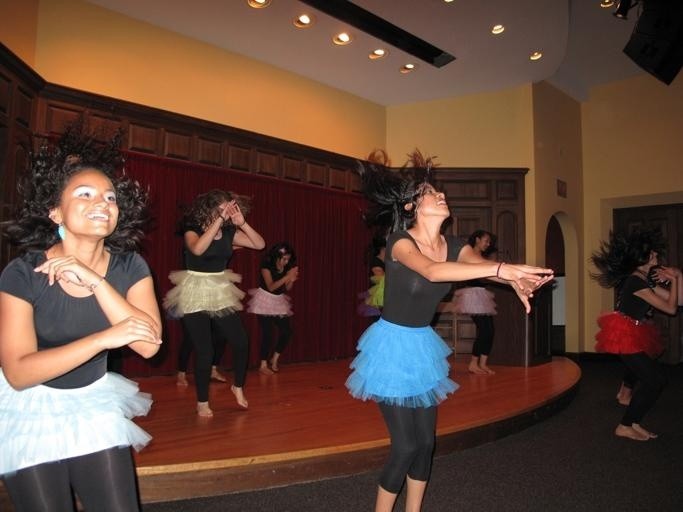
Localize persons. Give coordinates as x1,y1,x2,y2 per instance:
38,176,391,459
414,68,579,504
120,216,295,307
0,106,164,511
163,187,266,418
451,229,499,375
344,146,556,512
585,225,683,443
176,316,228,386
354,236,386,325
246,242,300,376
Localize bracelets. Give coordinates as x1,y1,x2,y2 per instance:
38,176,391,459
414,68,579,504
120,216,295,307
88,277,106,293
239,221,246,226
220,215,226,222
497,263,502,280
